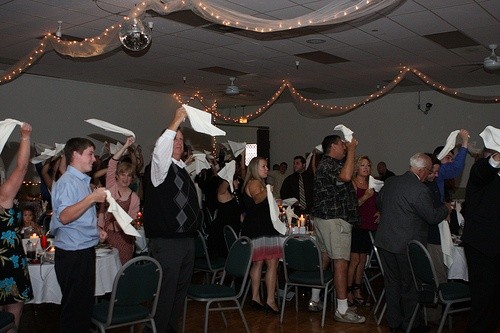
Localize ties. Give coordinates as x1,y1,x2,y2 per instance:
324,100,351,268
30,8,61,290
298,173,306,210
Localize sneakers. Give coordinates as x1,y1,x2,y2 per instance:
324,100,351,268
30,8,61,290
333,307,366,324
308,298,323,311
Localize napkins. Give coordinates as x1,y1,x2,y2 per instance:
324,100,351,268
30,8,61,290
0,101,500,267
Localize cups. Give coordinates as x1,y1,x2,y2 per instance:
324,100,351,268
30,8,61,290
450,234,463,247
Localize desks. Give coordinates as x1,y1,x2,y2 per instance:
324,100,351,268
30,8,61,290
25,247,123,306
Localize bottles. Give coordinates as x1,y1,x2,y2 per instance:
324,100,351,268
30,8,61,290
459,221,464,234
20,225,56,264
279,205,313,234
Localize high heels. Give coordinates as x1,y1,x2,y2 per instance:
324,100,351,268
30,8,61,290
249,300,282,314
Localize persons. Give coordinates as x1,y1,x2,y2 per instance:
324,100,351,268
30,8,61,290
309,134,366,323
89,136,144,266
49,137,108,333
16,147,64,255
141,104,200,333
374,161,395,181
345,155,380,307
377,126,500,333
280,156,312,223
0,118,34,333
187,143,288,314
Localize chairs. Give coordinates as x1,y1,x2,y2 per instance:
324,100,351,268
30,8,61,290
91,256,163,333
280,233,338,327
363,229,387,326
406,240,471,333
182,225,279,333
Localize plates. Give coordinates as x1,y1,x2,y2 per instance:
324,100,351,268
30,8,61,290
95,248,112,256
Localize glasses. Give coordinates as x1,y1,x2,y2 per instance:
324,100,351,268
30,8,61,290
425,166,433,174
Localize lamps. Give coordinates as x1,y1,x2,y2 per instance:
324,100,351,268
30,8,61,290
118,13,153,52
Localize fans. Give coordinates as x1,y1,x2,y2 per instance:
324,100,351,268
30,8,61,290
448,44,500,73
203,78,258,97
